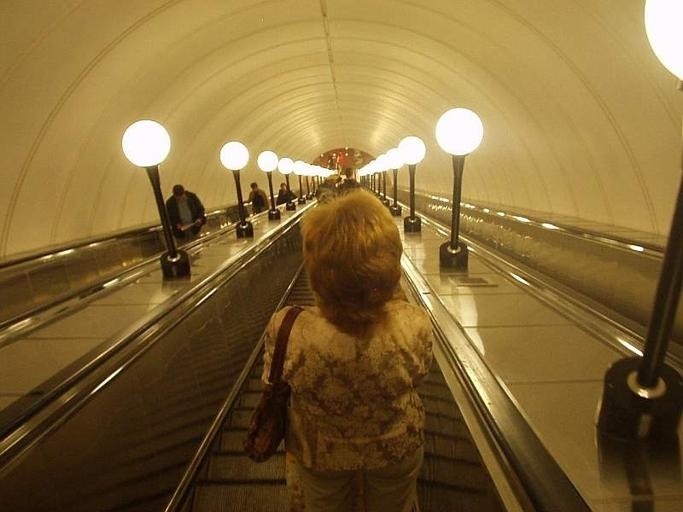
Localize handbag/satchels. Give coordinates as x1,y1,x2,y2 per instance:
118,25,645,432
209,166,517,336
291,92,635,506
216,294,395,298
242,385,289,462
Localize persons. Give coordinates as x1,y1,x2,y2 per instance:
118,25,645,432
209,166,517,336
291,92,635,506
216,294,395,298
166,185,206,247
261,190,433,512
276,183,297,205
242,183,269,214
316,167,360,202
